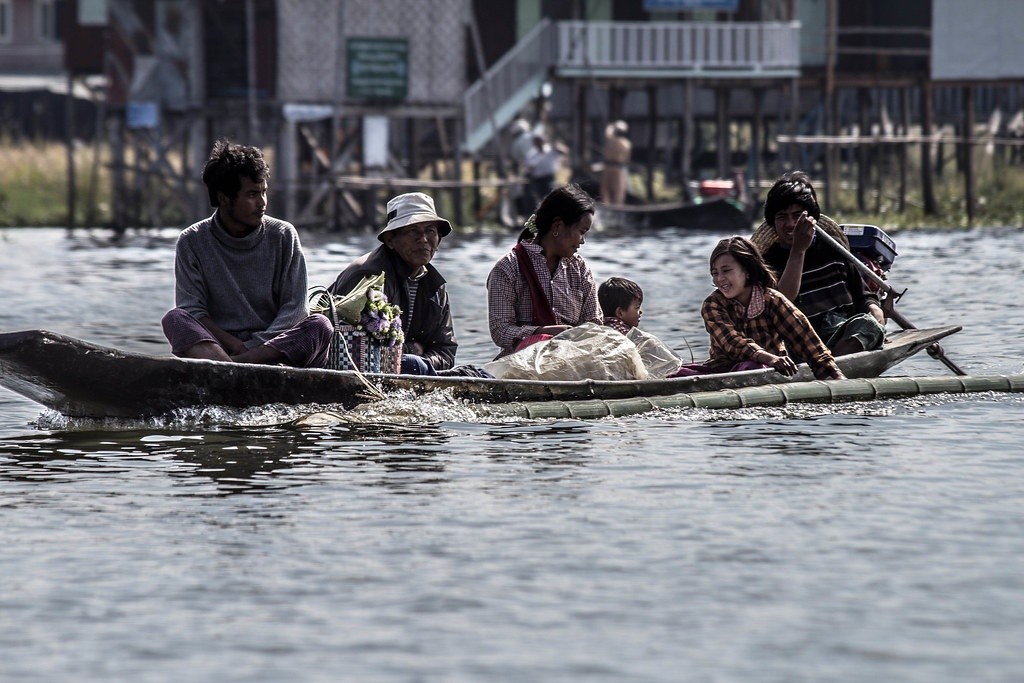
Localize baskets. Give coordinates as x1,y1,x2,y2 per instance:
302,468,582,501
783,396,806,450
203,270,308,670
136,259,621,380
308,286,403,374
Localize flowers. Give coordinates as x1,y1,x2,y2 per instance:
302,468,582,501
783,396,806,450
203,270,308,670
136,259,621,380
360,287,406,347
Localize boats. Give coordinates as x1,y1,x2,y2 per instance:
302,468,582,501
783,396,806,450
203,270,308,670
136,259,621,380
1,323,1024,416
503,200,754,238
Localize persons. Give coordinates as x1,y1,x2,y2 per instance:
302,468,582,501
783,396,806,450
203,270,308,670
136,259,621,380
508,117,557,215
668,237,847,379
162,138,334,368
93,11,194,197
486,182,604,362
601,120,632,206
761,171,885,357
598,277,643,336
317,192,458,375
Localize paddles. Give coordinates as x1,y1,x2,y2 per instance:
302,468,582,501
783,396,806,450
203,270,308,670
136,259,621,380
816,224,967,376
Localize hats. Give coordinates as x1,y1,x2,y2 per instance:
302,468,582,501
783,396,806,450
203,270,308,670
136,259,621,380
376,192,451,242
